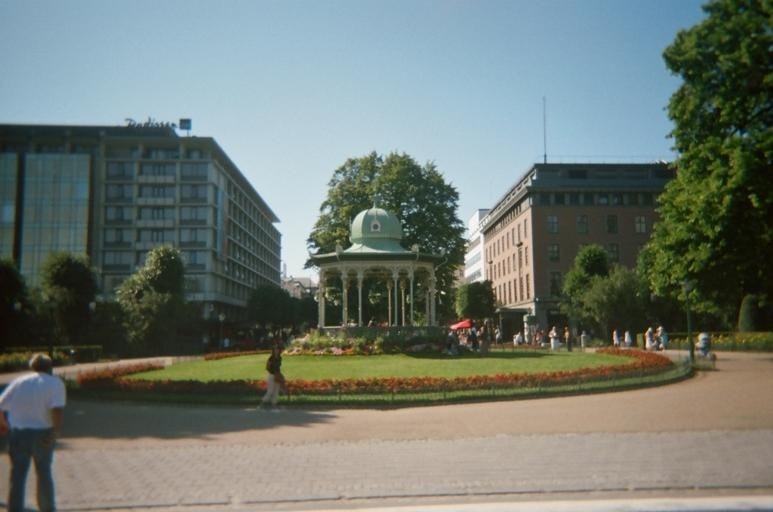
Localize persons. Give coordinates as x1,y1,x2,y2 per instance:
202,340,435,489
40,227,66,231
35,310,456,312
221,326,298,355
654,326,668,352
549,324,560,352
444,318,508,355
563,325,575,354
580,329,587,351
515,331,523,345
611,328,619,347
511,335,518,349
644,327,657,352
623,328,633,347
366,315,377,328
0,350,66,512
255,343,282,414
